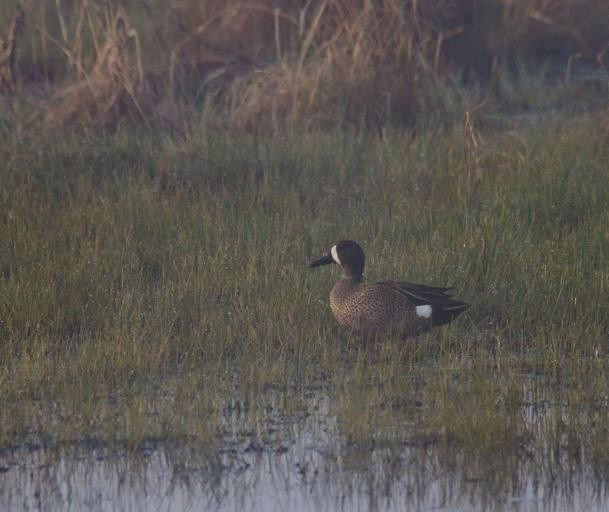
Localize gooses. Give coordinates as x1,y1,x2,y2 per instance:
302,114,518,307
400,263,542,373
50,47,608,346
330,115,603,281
308,240,472,343
